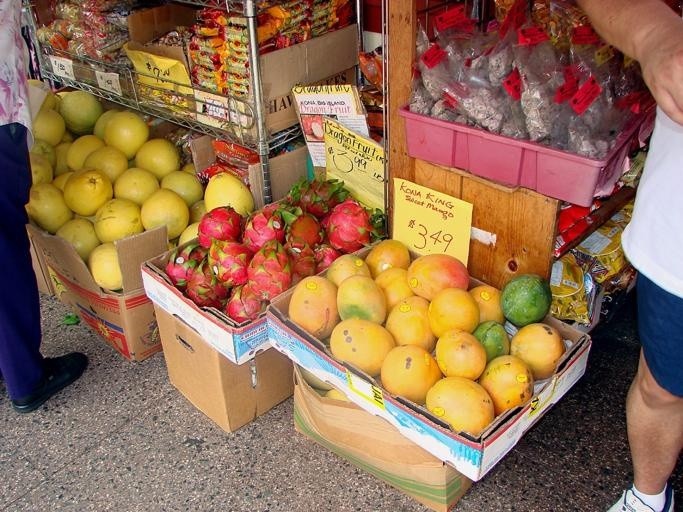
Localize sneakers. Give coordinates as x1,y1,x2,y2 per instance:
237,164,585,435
606,482,674,512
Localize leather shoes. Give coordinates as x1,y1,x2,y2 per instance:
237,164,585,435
12,351,89,414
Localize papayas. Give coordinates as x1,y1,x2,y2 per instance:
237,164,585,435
23,80,254,292
287,238,566,437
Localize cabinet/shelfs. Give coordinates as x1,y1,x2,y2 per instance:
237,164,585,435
23,0,364,211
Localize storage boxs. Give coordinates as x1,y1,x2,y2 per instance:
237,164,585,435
143,120,312,208
28,195,594,512
122,6,358,141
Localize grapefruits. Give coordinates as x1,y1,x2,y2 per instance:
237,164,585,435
23,80,254,292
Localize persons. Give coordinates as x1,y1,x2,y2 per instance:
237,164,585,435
573,0,683,512
0,1,87,412
